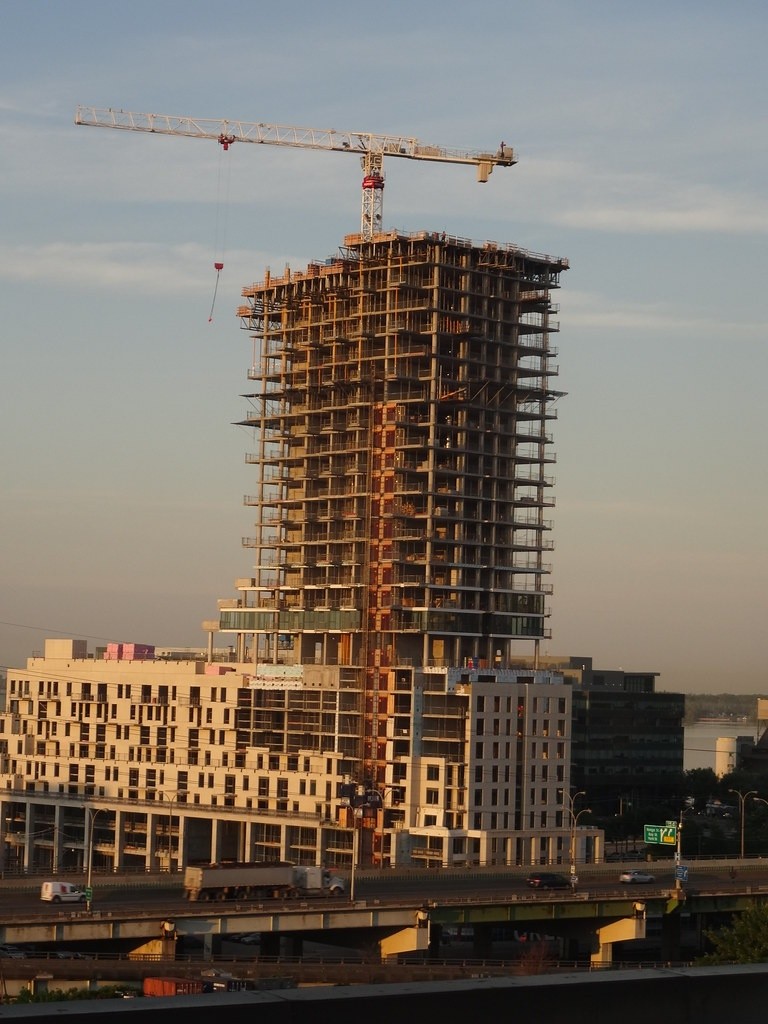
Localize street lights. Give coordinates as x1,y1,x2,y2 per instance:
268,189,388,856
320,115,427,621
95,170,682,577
368,788,400,870
728,789,759,859
559,789,585,863
340,802,370,902
80,803,109,913
561,807,592,892
674,806,694,890
147,787,191,874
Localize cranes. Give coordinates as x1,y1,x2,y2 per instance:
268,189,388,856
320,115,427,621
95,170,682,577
69,96,520,238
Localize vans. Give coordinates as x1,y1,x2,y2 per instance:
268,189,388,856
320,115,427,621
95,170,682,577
41,880,86,904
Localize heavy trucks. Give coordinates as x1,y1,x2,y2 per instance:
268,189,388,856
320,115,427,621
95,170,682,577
181,860,345,902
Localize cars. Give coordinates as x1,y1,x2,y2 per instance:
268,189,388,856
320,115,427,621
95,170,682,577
526,871,572,891
618,869,655,885
